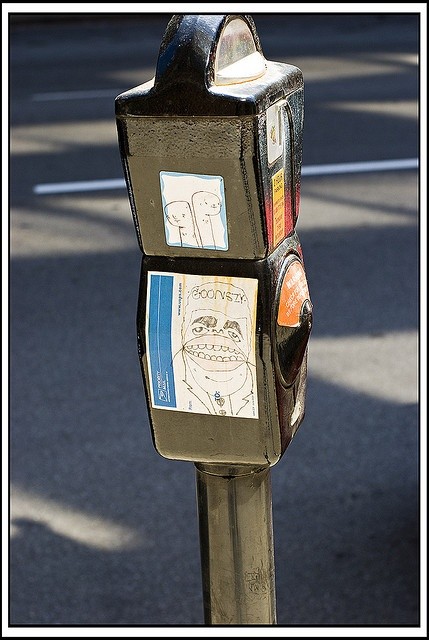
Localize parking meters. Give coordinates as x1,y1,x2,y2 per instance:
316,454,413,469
114,14,313,627
